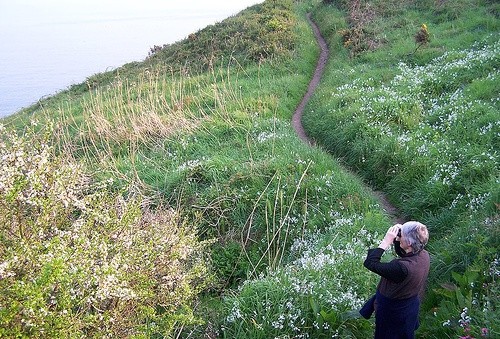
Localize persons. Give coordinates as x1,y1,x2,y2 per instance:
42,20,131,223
362,221,431,339
